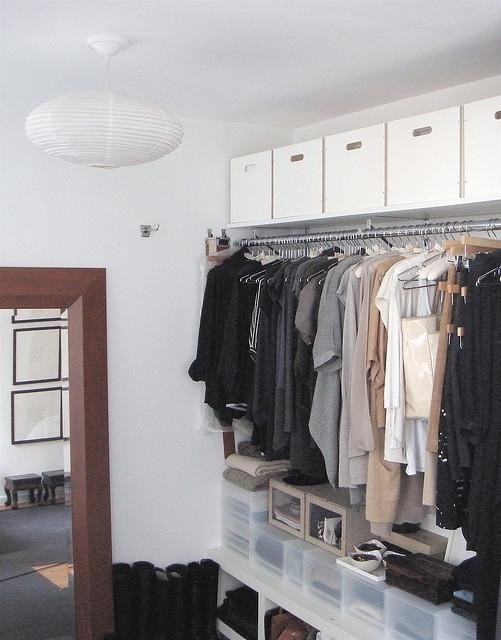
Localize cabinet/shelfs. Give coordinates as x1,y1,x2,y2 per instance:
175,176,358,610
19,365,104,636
230,94,501,223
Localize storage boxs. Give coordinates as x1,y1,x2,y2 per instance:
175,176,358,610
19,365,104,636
211,476,477,639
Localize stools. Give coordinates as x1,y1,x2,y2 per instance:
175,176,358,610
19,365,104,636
3,468,64,511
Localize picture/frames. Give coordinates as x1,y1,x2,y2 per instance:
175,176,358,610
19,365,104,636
11,325,64,445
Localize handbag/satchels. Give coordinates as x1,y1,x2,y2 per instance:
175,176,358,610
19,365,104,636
402,271,445,419
264,605,317,639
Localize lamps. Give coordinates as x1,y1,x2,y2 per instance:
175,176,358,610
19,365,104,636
23,32,185,169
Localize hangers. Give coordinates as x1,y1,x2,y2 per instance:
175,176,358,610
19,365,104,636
238,215,501,292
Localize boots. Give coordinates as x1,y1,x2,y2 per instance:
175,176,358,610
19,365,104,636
113,558,222,639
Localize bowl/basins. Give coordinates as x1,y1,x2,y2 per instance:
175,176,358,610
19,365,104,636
348,538,408,573
